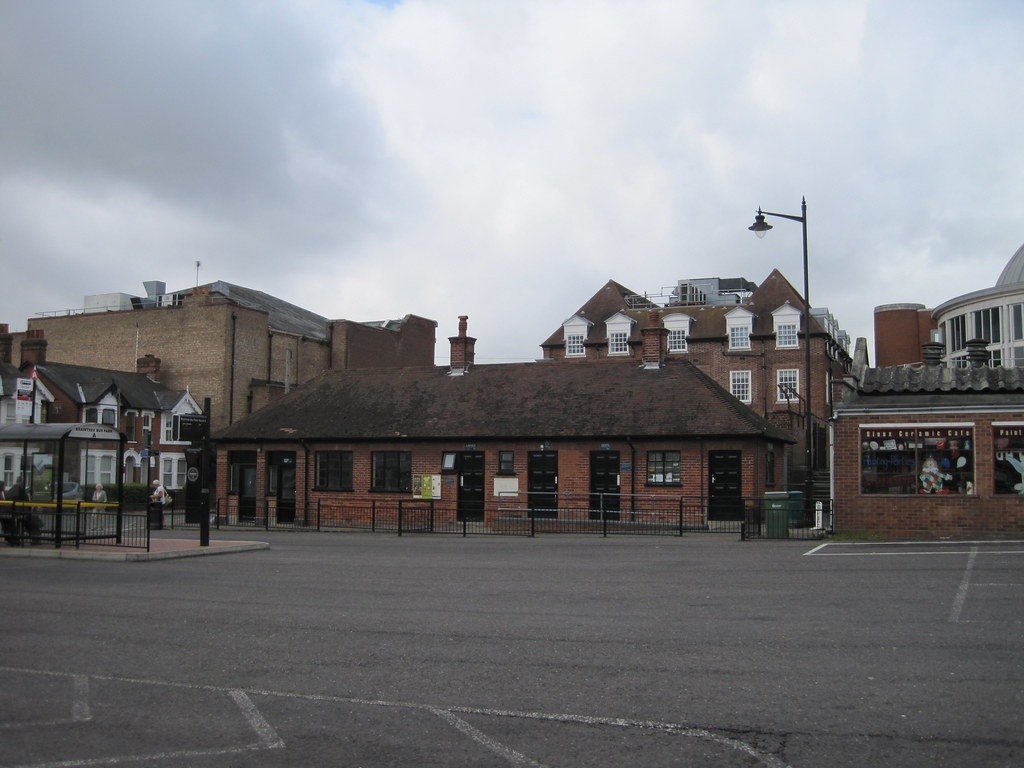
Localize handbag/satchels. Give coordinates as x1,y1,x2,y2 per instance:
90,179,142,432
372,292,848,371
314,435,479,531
164,490,172,507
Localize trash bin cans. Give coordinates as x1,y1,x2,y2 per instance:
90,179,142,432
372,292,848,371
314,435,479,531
148,502,163,530
764,491,803,540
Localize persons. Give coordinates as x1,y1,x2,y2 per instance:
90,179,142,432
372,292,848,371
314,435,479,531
91,483,107,531
0,475,41,546
150,480,166,527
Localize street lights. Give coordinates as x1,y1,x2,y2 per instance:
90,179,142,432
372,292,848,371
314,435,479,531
748,195,813,526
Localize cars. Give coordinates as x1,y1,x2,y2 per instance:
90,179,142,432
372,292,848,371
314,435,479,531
43,482,83,499
